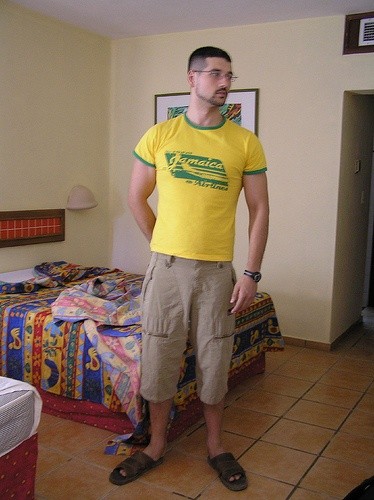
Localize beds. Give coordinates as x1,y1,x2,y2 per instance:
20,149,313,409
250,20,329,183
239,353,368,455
0,261,284,456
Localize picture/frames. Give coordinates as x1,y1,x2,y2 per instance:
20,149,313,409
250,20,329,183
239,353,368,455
154,88,259,137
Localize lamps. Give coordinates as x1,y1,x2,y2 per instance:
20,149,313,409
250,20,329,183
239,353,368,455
67,185,97,210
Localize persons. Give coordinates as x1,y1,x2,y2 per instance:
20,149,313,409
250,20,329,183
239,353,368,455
108,46,269,491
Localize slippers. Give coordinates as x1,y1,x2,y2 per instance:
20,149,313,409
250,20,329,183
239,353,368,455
108,450,165,485
208,453,247,491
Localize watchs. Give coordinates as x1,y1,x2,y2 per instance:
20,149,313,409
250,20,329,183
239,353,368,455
244,270,261,283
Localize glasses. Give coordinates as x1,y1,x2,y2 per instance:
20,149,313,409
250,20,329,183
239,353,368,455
192,69,238,82
